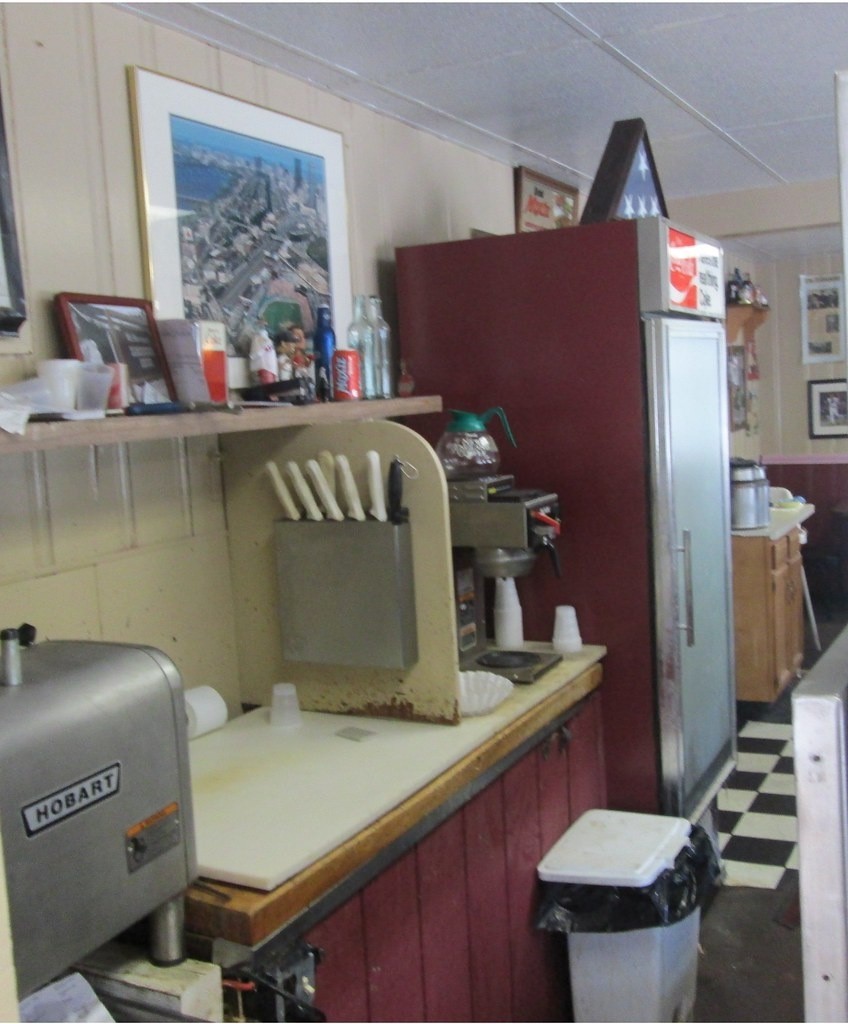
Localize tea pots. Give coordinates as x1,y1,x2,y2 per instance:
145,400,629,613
438,405,515,477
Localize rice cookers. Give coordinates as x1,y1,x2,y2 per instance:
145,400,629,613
729,460,770,529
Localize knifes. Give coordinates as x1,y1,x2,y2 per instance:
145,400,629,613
268,452,412,526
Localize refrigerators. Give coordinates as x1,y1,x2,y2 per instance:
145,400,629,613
395,225,733,824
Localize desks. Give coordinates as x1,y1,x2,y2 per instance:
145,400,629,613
122,641,612,1023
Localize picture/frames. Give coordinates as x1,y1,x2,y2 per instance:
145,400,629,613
810,378,848,438
799,273,846,366
0,94,28,336
54,291,177,407
727,346,748,431
125,63,359,399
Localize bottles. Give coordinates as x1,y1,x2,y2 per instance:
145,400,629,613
347,294,377,399
313,304,338,399
256,329,278,383
369,298,391,399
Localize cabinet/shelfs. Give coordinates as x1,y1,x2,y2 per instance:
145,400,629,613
729,504,817,710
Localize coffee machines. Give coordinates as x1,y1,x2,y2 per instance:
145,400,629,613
444,474,563,682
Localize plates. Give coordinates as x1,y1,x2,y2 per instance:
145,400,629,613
453,671,512,716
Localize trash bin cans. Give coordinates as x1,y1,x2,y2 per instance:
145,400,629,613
532,808,720,1022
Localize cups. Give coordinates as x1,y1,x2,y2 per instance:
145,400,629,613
551,605,585,653
268,683,301,725
494,575,524,652
75,365,114,412
38,359,78,408
